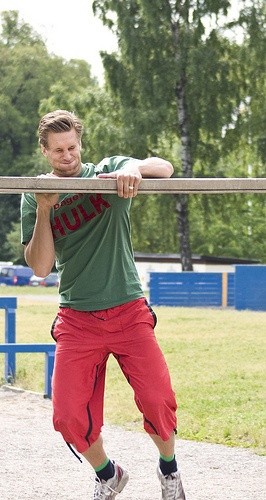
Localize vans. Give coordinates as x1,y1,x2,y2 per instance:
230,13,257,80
0,267,33,286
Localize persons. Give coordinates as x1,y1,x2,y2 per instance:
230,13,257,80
20,109,187,500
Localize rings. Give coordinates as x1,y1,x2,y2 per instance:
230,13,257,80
129,186,135,190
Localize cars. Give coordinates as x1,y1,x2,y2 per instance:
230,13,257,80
29,273,58,287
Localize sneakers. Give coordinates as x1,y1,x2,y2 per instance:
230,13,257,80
157,464,186,500
94,461,129,500
